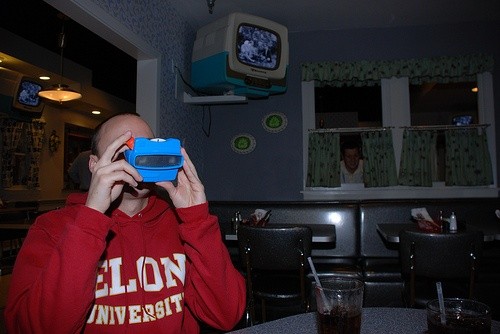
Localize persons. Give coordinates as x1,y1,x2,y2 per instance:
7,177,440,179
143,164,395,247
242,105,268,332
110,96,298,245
19,82,41,104
453,118,471,125
340,143,363,183
239,25,277,63
61,150,92,191
4,110,246,334
65,161,73,172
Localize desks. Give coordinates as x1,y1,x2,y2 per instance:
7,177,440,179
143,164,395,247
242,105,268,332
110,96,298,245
377,222,492,243
225,224,337,243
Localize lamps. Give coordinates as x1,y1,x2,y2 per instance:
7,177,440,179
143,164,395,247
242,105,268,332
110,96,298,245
38,29,82,105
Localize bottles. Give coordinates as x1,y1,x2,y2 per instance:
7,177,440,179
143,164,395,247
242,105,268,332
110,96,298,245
449,212,457,233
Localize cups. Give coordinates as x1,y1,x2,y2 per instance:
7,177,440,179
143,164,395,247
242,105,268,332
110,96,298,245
425,298,492,334
313,278,364,334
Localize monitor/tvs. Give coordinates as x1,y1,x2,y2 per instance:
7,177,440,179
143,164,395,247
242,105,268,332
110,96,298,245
190,13,290,86
10,75,48,114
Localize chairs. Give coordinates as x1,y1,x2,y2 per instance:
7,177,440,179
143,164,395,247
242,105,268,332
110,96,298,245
399,229,480,307
237,225,313,328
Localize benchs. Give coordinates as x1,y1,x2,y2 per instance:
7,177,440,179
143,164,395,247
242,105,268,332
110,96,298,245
208,196,500,306
